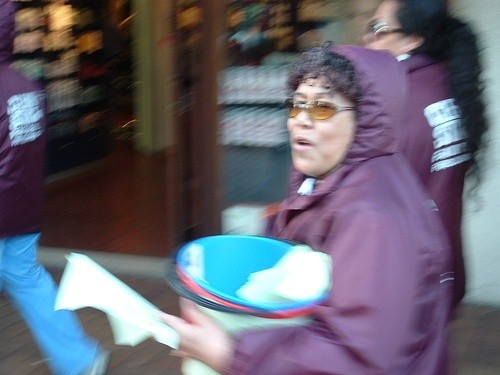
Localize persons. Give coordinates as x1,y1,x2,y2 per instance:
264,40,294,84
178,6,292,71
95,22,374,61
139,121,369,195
160,46,451,375
360,0,485,312
0,0,112,375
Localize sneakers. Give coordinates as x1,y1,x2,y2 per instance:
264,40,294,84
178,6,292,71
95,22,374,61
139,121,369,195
82,346,113,375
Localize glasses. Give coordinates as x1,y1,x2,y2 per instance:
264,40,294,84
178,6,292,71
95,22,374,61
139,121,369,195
366,15,405,40
282,97,357,122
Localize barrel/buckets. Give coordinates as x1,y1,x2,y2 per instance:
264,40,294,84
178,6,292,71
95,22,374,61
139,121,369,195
171,236,332,374
